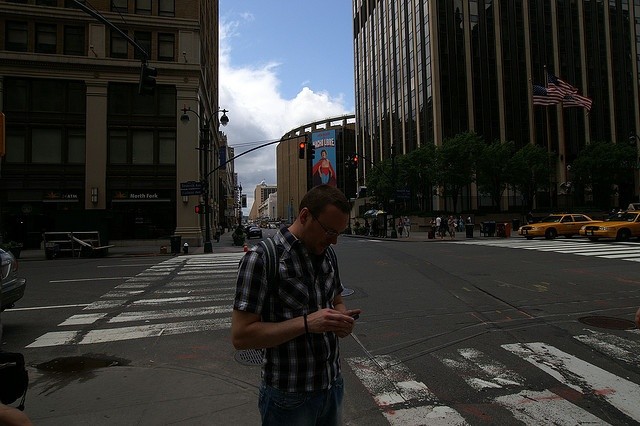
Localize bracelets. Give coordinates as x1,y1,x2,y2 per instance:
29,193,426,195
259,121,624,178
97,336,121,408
304,313,309,335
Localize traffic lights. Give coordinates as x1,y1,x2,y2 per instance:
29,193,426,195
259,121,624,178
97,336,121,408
195,206,204,214
308,143,315,159
139,63,157,96
299,142,305,159
352,157,358,168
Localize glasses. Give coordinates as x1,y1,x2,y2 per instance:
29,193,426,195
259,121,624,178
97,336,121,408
312,211,345,238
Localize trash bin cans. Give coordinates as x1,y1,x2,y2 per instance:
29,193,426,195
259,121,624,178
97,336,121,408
170,235,181,252
466,223,475,238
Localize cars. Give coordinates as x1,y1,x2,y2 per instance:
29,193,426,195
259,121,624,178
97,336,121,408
518,213,604,241
579,211,640,241
248,227,262,239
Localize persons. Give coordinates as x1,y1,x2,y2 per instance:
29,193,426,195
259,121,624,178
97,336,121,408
377,214,385,238
526,211,533,225
232,185,361,425
448,216,456,241
403,216,411,239
1,351,33,426
440,215,448,240
398,218,403,238
458,215,465,232
430,216,437,239
436,216,441,236
466,215,472,224
318,150,333,185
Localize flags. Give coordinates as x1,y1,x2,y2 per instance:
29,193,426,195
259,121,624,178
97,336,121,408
561,92,593,117
533,84,564,106
546,69,579,100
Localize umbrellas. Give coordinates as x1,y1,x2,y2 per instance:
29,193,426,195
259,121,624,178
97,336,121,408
365,210,378,216
372,209,388,217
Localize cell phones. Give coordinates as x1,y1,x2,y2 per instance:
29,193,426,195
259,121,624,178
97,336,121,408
349,313,359,320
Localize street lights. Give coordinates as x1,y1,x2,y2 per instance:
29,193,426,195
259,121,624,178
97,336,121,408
180,108,229,253
